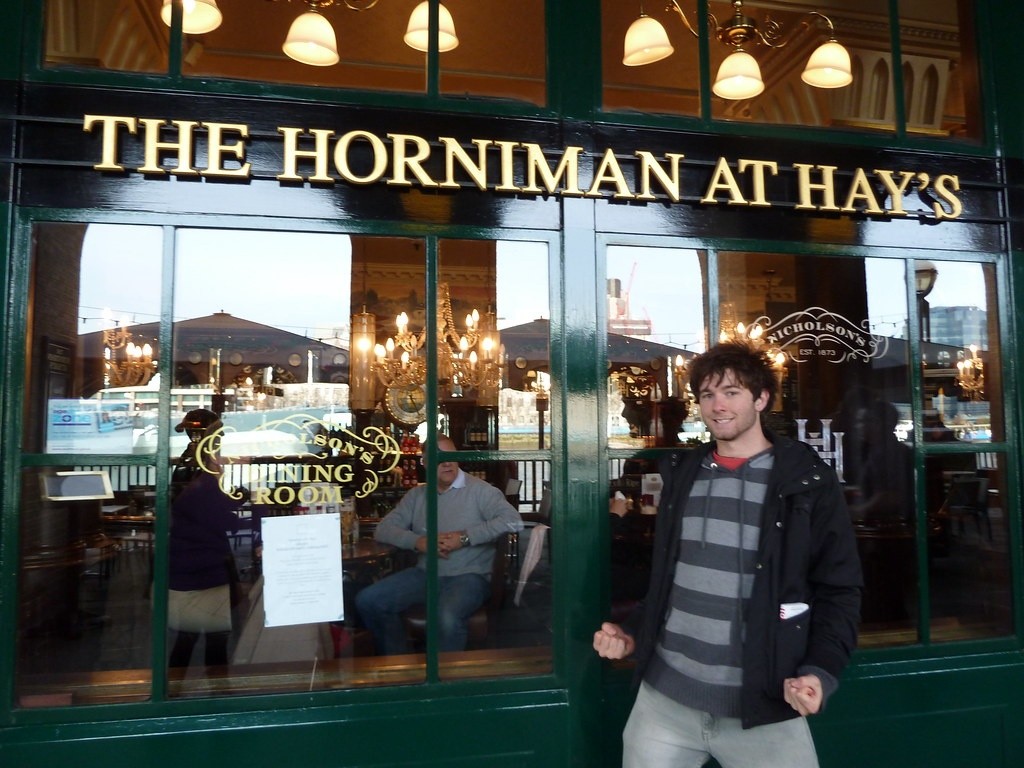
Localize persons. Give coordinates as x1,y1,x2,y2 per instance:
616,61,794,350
172,405,337,701
162,410,272,699
351,432,525,661
592,337,864,768
603,360,969,639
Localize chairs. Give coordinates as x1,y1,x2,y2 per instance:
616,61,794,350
926,469,990,558
101,485,157,581
504,479,554,572
401,532,512,649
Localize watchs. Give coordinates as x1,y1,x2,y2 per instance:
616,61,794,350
459,530,471,548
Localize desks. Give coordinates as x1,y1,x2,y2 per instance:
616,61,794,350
610,497,658,545
359,516,383,540
102,505,130,516
342,540,400,575
126,491,157,508
237,500,292,546
851,502,941,552
18,540,117,660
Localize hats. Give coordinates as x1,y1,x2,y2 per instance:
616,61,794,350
175,409,219,443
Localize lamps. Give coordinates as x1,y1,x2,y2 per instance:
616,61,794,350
101,327,158,386
621,0,854,101
674,252,787,418
374,239,506,390
160,0,460,67
957,343,986,394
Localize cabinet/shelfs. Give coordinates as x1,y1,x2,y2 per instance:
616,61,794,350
355,398,498,506
